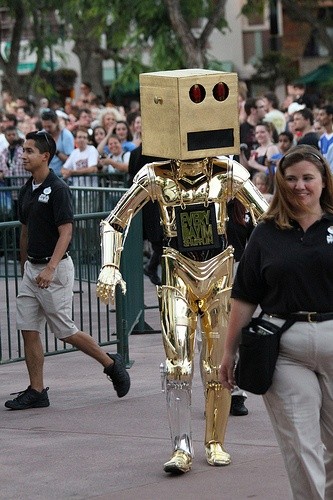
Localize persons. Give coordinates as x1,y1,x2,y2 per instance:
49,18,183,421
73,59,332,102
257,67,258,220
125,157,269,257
0,82,332,288
221,146,333,500
4,130,131,411
92,66,272,476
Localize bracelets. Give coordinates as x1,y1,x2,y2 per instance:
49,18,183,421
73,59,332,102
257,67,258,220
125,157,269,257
56,150,60,156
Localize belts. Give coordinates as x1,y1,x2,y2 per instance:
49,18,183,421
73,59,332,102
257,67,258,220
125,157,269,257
27,251,70,264
265,312,332,322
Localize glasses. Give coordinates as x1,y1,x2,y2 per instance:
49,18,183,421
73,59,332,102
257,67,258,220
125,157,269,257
36,130,49,145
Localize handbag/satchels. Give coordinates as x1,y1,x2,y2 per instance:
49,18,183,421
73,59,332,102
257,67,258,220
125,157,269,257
234,318,281,395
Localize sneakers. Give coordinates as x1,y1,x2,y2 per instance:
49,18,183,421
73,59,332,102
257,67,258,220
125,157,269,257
4,385,49,408
102,352,130,398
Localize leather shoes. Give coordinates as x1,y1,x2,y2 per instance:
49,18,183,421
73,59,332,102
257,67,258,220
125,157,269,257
230,395,248,416
